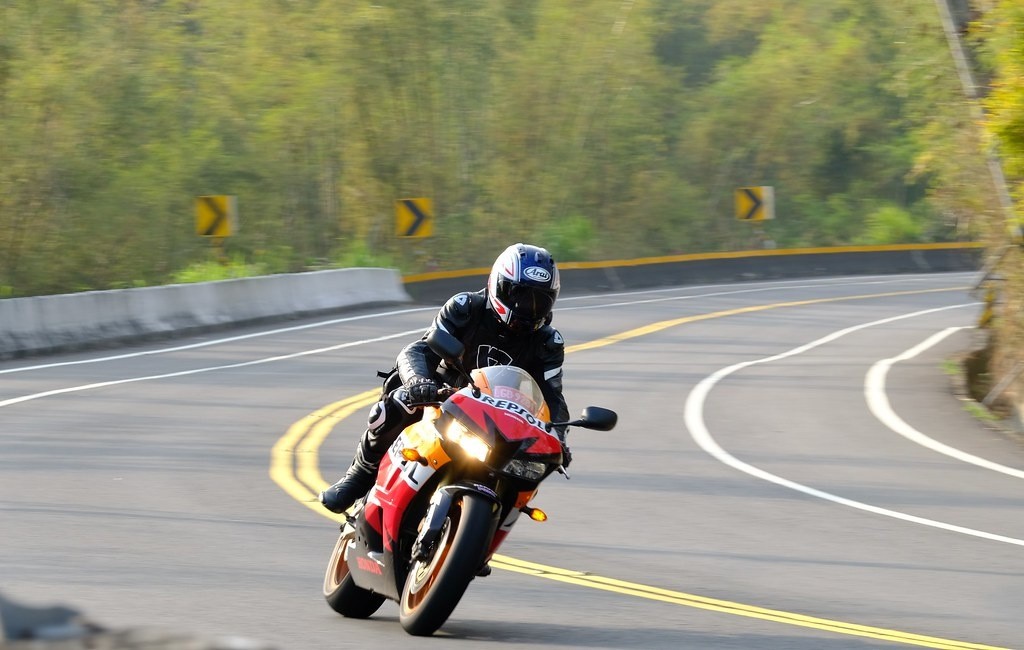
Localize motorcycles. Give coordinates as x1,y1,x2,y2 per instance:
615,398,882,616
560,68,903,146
322,329,617,637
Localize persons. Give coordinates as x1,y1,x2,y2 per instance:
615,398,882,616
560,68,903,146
319,242,572,513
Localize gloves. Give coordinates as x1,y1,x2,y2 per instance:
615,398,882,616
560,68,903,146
562,442,573,474
406,375,438,403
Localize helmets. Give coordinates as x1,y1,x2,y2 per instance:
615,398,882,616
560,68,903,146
487,242,561,332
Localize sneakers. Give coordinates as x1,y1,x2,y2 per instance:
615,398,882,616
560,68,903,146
477,563,492,577
319,431,377,513
